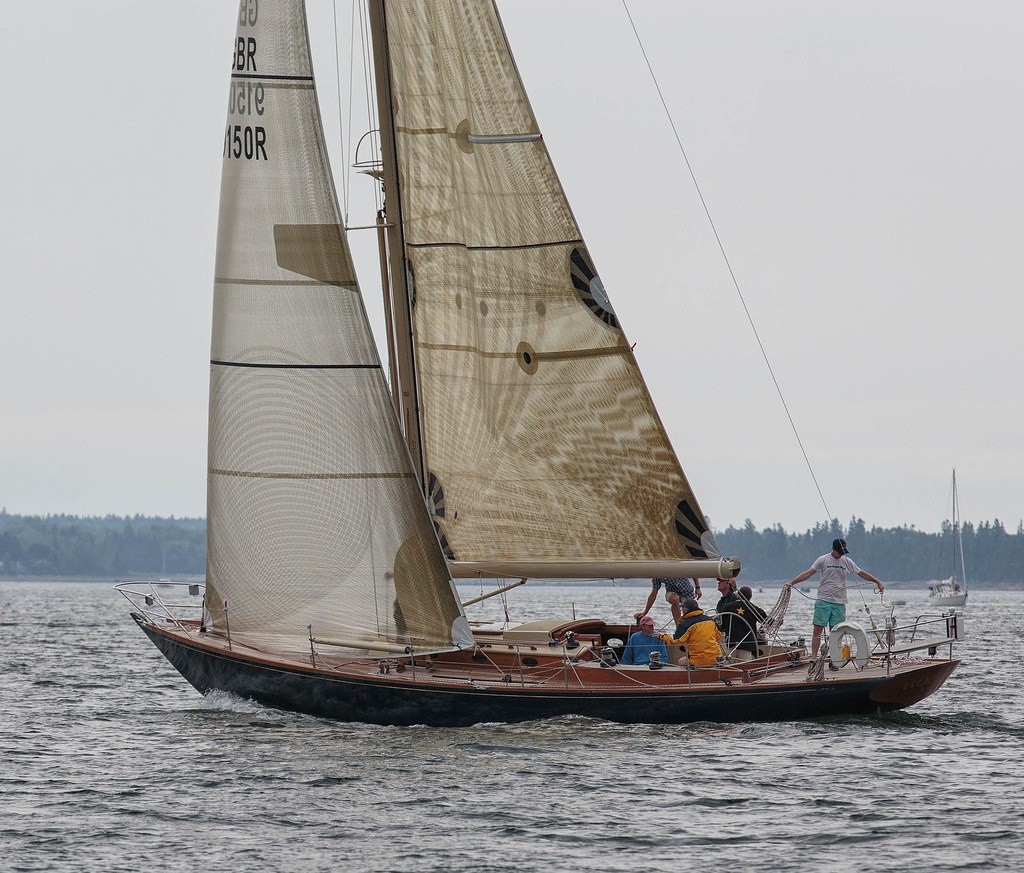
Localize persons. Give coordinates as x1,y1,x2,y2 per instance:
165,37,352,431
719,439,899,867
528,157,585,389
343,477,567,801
633,575,703,631
652,601,726,666
783,538,884,669
713,578,777,665
620,616,669,666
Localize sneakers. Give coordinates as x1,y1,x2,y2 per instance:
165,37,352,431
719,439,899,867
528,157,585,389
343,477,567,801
808,658,818,673
828,660,840,671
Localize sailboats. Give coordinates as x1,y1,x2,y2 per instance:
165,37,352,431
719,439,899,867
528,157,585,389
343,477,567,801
928,467,967,607
158,543,173,589
108,2,967,728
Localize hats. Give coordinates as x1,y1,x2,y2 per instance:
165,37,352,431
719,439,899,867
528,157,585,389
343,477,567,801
640,616,658,625
833,539,849,554
738,586,753,600
678,599,699,609
717,577,737,587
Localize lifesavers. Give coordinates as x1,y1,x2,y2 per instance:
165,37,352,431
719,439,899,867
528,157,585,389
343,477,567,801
829,622,869,669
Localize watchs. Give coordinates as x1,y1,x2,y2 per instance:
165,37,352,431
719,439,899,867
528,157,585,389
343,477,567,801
695,585,701,590
